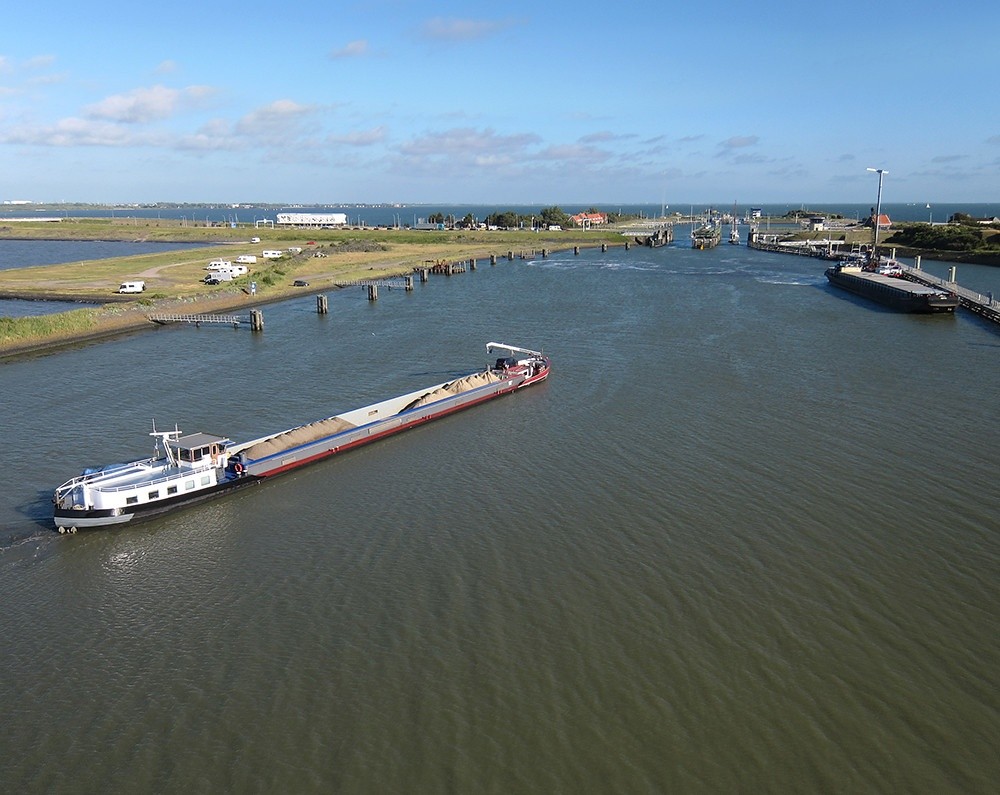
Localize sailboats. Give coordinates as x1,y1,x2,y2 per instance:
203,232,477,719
690,200,742,250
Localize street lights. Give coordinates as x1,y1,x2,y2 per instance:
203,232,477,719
866,167,890,244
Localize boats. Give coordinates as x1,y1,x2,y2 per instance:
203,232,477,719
824,249,962,314
50,339,552,535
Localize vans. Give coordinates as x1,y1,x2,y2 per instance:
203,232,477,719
235,254,257,264
549,225,562,232
262,250,283,261
203,260,248,282
251,237,261,244
118,280,147,294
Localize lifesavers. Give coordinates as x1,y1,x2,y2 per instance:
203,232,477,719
234,463,242,472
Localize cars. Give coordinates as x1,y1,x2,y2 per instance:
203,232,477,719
307,241,316,245
205,279,220,285
294,280,309,287
